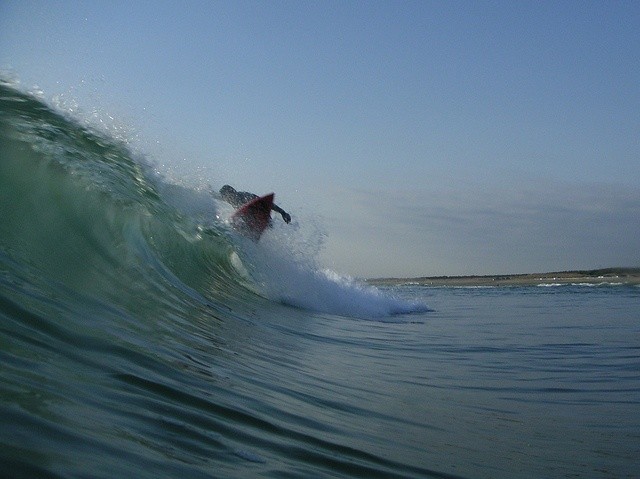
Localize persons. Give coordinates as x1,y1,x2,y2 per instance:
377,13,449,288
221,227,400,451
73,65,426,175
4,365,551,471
212,185,291,229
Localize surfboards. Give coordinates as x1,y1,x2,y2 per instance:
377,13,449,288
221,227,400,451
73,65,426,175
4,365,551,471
229,192,275,244
